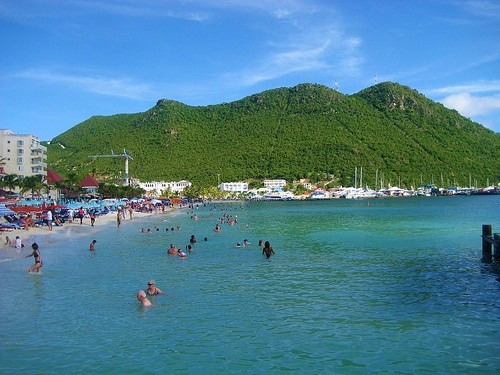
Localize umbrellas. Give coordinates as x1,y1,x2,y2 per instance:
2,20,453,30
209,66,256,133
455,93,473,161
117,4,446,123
81,197,192,208
0,199,83,216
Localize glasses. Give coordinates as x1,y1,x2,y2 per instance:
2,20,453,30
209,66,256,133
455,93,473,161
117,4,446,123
148,283,153,285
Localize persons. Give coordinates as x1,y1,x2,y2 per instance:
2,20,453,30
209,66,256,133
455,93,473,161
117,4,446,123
67,207,75,223
144,280,163,295
26,241,43,272
90,240,96,251
90,208,95,226
168,243,193,259
237,239,275,258
137,290,151,307
5,236,25,248
78,207,85,224
189,235,196,243
187,202,249,232
44,208,53,230
135,201,186,213
139,224,180,233
117,201,134,227
